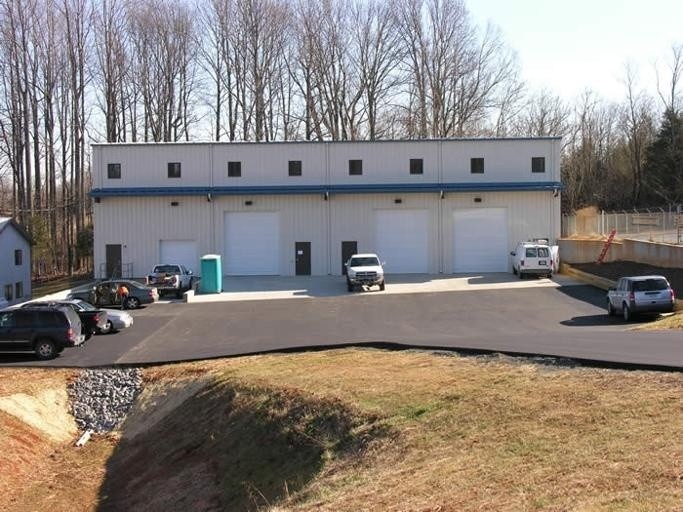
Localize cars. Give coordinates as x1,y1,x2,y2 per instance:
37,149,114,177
64,278,159,310
22,297,134,333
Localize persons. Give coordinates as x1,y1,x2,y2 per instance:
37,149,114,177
119,286,129,309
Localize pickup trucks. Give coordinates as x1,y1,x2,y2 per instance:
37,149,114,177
21,303,108,340
143,263,194,300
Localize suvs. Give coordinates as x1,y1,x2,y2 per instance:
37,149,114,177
344,252,385,292
509,241,553,279
606,274,674,323
0,304,86,360
